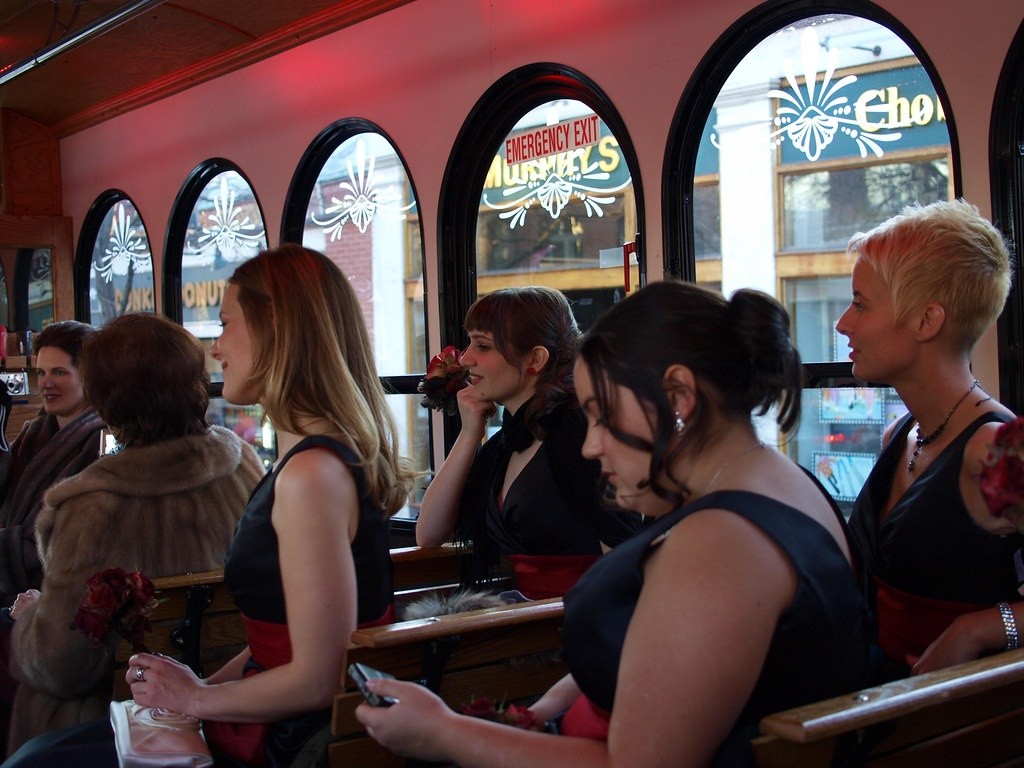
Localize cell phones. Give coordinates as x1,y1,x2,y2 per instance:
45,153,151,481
98,428,127,458
345,663,401,707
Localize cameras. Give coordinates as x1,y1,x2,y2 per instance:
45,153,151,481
0,368,30,395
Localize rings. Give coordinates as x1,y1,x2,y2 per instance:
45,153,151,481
136,665,148,680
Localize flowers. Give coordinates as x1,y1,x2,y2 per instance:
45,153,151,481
459,691,549,734
978,416,1024,524
72,565,158,661
416,344,492,421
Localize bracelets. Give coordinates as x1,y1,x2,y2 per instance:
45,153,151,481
999,601,1018,651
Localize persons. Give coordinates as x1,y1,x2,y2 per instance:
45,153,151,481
0,239,405,768
404,289,642,635
5,311,264,755
0,316,110,625
353,280,868,768
835,196,1024,672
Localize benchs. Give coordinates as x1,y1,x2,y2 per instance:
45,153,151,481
749,648,1024,768
107,540,480,715
323,591,574,768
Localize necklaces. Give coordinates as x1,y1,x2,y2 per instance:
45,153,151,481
906,381,979,474
706,438,765,493
